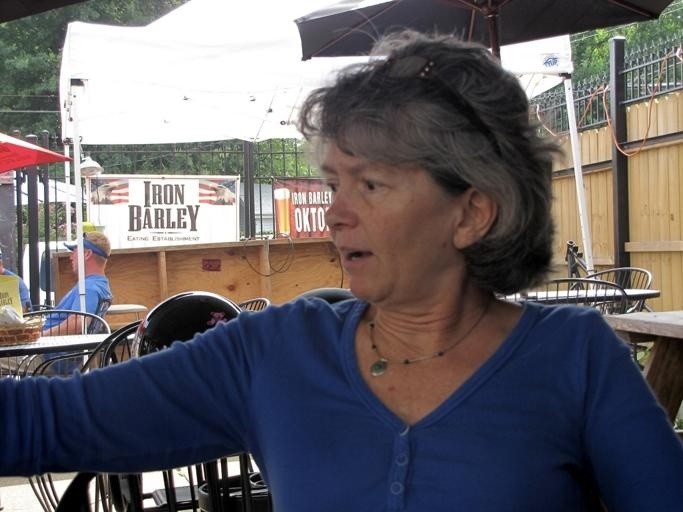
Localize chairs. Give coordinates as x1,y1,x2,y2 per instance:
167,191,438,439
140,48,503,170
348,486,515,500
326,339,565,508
569,267,652,362
22,309,111,381
10,300,111,380
105,325,162,373
196,297,269,496
494,277,628,323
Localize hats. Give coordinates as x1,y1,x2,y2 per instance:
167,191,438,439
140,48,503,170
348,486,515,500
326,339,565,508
64,239,109,259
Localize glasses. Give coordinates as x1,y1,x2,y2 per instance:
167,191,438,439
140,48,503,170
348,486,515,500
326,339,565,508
382,52,505,160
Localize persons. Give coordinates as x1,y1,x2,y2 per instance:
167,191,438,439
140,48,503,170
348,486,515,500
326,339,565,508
0,30,683,511
0,231,113,377
0,247,32,316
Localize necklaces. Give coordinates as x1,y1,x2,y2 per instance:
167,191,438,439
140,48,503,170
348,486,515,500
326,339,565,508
364,298,495,378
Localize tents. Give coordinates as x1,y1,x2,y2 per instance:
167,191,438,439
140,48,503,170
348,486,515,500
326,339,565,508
59,0,597,378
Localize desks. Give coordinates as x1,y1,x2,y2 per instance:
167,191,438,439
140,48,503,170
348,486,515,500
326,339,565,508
1,334,136,385
502,289,660,316
2,301,147,341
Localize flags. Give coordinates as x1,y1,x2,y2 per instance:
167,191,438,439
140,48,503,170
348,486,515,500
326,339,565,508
91,182,129,204
199,178,237,206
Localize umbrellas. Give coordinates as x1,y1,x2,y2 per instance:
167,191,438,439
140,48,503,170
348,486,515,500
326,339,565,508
0,131,73,173
292,0,680,60
